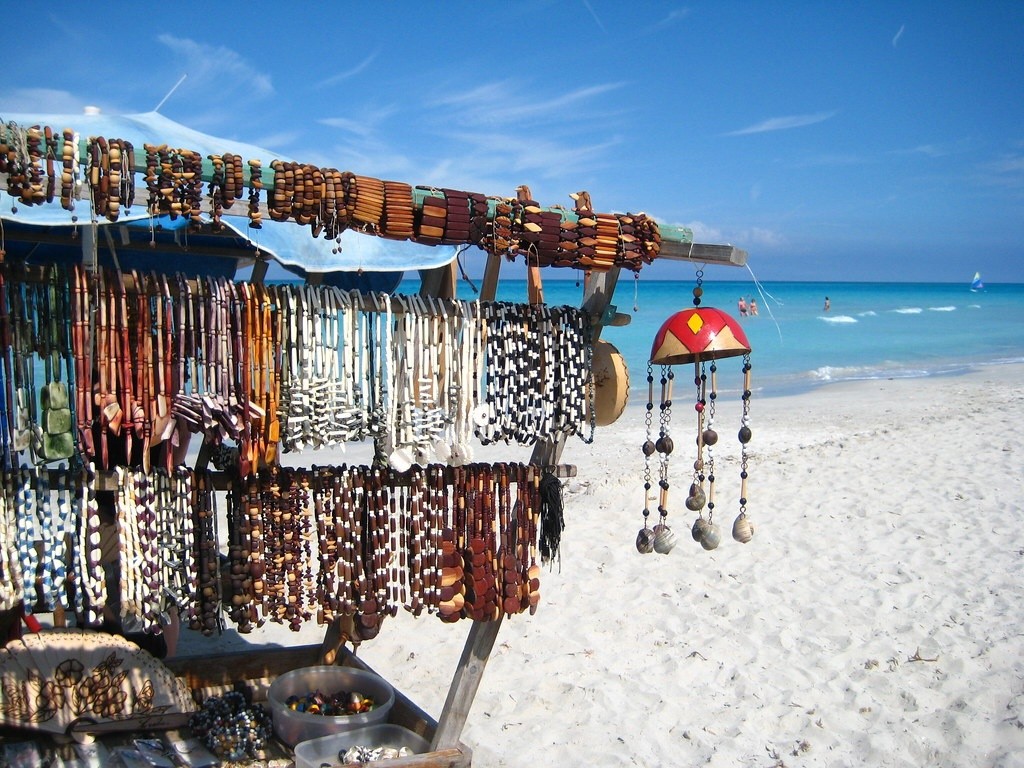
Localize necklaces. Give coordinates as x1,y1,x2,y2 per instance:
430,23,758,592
0,246,597,637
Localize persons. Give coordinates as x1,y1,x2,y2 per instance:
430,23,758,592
823,297,831,314
751,299,757,315
738,296,749,317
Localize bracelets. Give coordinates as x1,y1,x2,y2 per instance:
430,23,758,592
180,677,277,764
1,114,665,284
